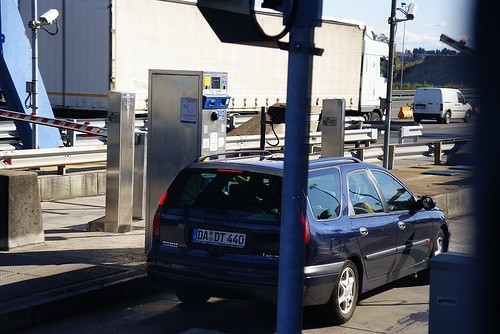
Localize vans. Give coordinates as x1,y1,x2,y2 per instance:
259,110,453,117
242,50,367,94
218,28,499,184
411,88,473,124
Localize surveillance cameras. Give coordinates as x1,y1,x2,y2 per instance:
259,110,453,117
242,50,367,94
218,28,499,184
39,8,59,24
406,3,417,20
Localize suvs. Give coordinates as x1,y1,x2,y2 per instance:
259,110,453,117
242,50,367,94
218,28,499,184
145,149,452,325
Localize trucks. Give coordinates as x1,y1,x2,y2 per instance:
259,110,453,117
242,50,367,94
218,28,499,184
17,0,392,133
